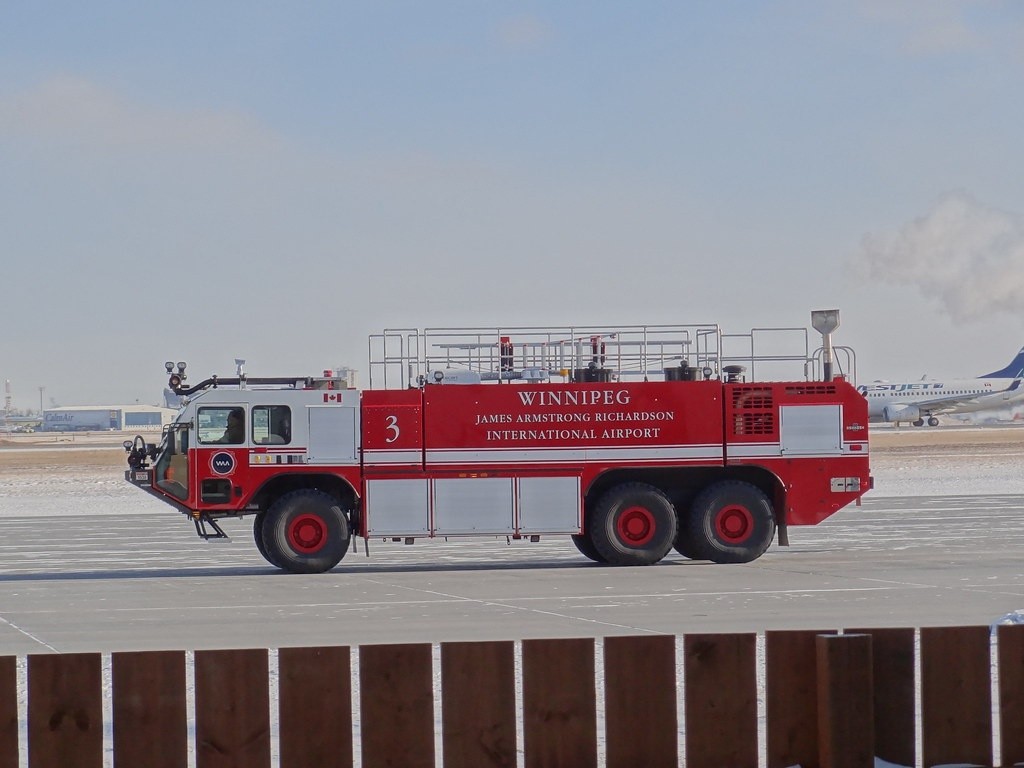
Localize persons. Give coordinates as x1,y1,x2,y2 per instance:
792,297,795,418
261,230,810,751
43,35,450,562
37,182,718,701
202,410,243,444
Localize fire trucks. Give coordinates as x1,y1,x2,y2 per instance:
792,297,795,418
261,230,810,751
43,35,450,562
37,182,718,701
123,304,878,573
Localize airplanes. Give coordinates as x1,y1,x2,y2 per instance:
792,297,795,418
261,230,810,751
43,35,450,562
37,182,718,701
852,348,1024,427
